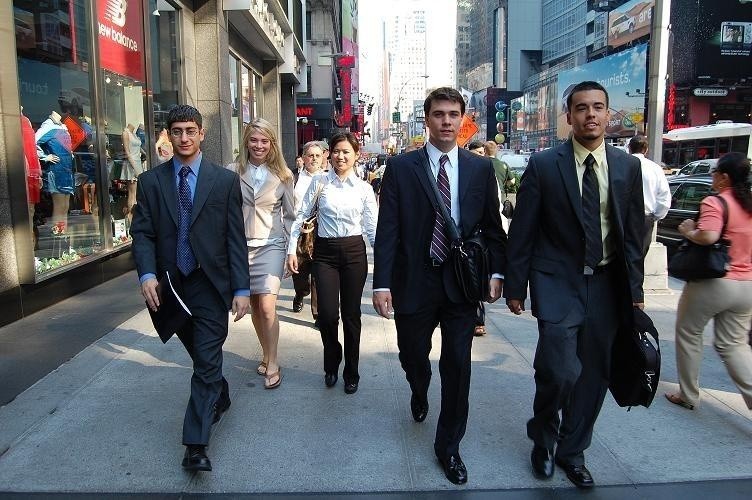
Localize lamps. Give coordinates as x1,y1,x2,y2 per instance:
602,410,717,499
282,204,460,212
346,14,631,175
626,89,648,98
297,117,308,126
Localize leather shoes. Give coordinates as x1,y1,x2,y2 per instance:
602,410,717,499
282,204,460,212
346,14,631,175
411,394,429,422
182,445,212,471
293,295,302,312
530,444,594,488
325,370,359,393
441,453,467,484
211,396,230,424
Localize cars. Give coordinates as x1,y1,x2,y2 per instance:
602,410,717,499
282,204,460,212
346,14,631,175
500,155,529,170
675,158,718,176
657,175,715,245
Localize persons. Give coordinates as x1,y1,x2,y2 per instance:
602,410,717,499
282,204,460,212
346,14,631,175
286,138,519,334
16,104,176,259
374,83,507,488
221,117,295,395
285,130,379,396
503,79,645,495
127,104,253,476
624,133,673,261
662,151,751,417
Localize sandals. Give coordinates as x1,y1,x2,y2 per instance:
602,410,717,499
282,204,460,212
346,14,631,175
474,327,487,336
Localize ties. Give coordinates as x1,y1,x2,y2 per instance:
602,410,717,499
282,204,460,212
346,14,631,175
432,154,451,263
582,153,603,268
176,166,198,276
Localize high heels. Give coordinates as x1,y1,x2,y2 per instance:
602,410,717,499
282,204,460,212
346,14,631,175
665,392,694,409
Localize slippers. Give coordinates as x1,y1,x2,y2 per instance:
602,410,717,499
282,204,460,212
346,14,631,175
257,361,281,388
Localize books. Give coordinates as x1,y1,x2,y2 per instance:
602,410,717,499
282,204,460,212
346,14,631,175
145,269,193,347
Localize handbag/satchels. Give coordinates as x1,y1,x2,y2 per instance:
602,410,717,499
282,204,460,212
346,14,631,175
670,235,730,278
456,224,490,303
297,217,317,261
609,304,661,406
502,200,514,219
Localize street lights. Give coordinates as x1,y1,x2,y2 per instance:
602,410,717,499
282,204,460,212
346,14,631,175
397,74,430,153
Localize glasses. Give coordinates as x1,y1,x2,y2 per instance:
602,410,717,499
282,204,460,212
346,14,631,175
170,130,197,136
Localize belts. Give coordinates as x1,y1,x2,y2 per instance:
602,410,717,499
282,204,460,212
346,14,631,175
581,265,616,275
426,260,446,266
